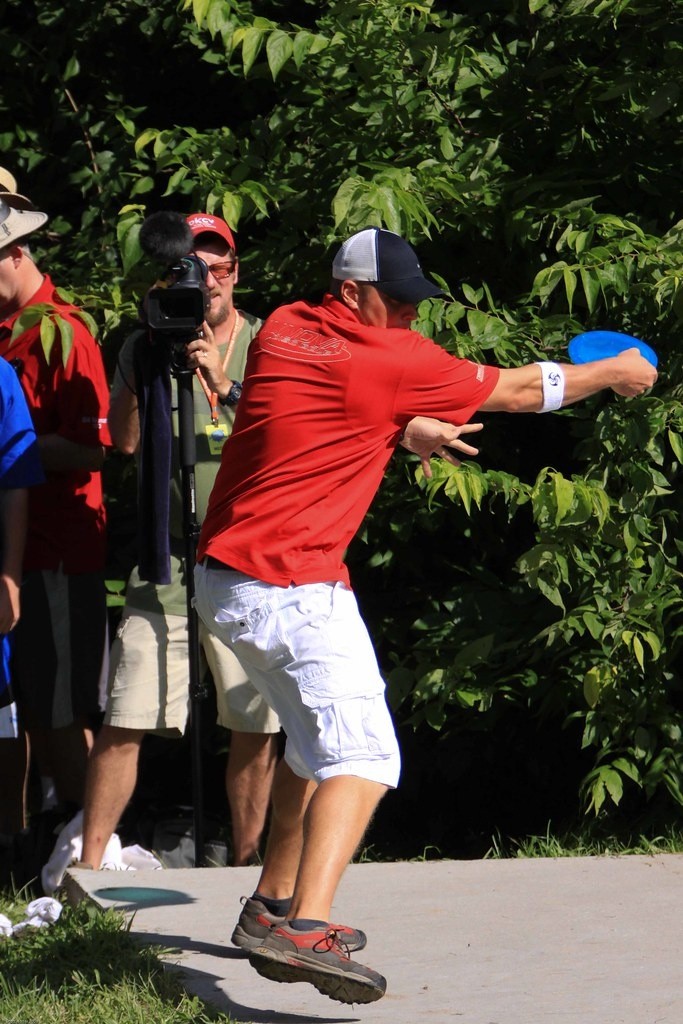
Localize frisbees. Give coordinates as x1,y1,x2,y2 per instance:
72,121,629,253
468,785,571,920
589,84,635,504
568,330,658,368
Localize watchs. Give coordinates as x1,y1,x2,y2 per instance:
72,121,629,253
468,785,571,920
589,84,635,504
218,380,242,407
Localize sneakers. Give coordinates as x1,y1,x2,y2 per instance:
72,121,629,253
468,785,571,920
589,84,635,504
250,921,387,1004
232,896,367,953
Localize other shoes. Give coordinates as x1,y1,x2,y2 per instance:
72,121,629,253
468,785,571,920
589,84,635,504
0,831,33,883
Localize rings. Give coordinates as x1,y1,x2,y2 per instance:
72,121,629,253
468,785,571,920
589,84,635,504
204,353,207,356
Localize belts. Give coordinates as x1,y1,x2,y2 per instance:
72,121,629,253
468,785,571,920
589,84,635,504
199,556,233,570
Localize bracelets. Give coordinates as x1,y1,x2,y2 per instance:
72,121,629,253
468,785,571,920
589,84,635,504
536,360,565,413
400,426,406,441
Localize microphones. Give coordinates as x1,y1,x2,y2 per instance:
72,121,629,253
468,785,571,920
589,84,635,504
137,211,194,267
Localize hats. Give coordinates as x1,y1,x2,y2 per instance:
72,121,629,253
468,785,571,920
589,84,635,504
333,227,454,303
187,213,235,254
0,198,49,249
0,166,34,211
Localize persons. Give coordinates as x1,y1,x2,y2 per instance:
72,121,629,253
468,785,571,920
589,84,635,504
0,168,112,898
65,212,265,872
193,229,657,1006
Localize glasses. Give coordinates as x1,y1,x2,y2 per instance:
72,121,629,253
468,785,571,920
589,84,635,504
208,256,235,279
